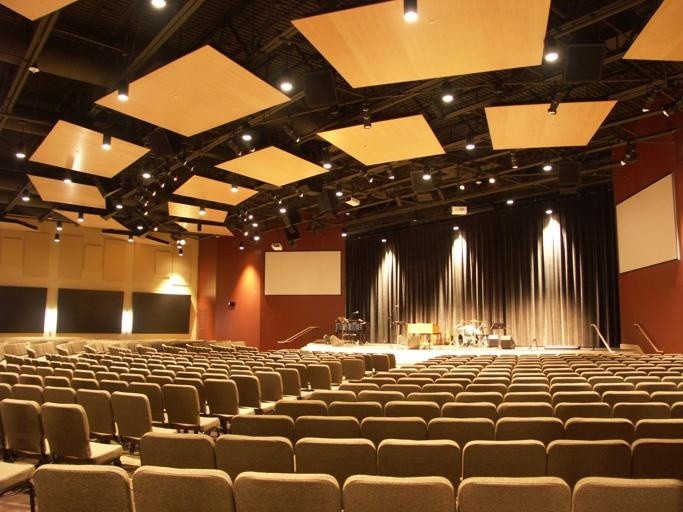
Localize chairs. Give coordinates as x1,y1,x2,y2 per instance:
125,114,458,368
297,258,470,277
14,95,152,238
0,338,683,512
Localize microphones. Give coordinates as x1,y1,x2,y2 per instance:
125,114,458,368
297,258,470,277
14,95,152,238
351,311,359,316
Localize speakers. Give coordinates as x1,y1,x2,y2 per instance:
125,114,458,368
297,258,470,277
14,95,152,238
147,128,178,160
282,207,300,227
489,334,499,346
562,42,603,85
93,176,123,199
558,161,581,183
500,335,516,348
302,68,338,108
317,190,337,212
411,169,435,193
284,227,301,244
408,335,420,349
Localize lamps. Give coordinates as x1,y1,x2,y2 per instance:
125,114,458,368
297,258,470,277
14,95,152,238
14,103,680,257
151,0,166,9
442,82,454,104
403,1,417,22
544,38,558,63
117,82,130,101
279,70,294,93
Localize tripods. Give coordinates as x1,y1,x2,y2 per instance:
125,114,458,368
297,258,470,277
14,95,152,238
340,323,355,348
